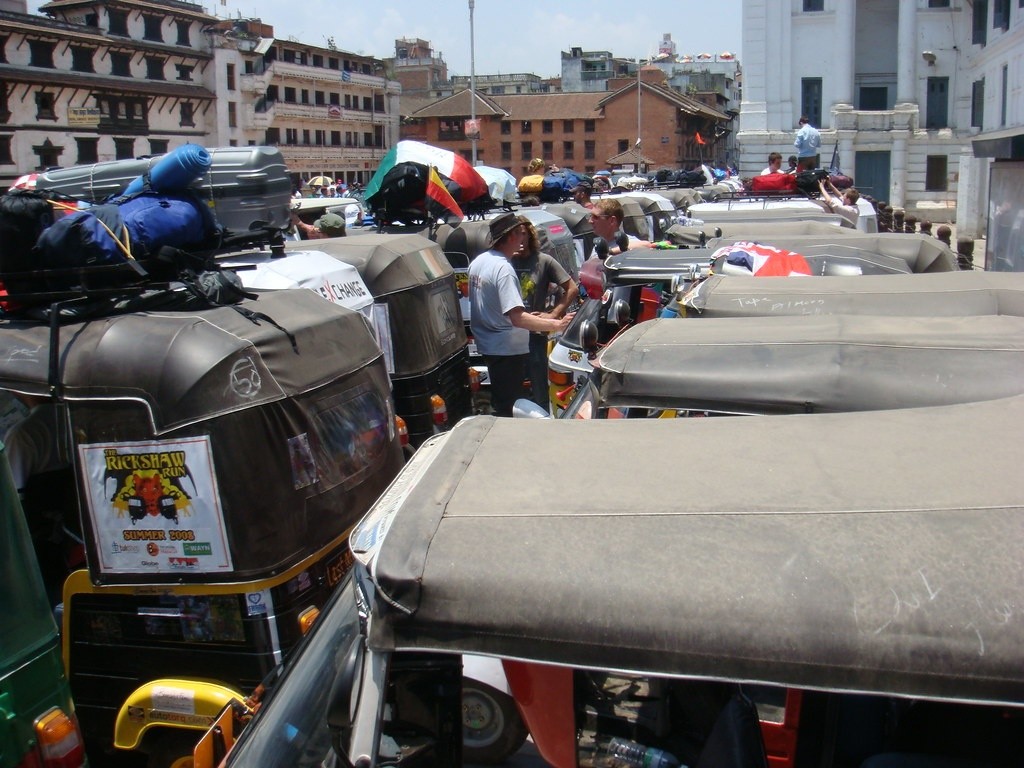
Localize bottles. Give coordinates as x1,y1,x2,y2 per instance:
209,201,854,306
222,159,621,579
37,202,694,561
654,241,677,250
660,295,678,318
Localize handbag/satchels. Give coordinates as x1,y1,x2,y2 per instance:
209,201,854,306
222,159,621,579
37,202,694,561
363,139,741,225
747,166,853,198
0,145,220,297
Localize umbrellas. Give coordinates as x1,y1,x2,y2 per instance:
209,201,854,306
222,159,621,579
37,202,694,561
721,51,735,62
678,55,692,63
307,175,332,186
697,53,711,61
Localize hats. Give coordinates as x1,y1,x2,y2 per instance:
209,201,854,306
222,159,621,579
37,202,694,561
313,213,347,237
483,213,532,251
568,185,590,193
345,204,360,224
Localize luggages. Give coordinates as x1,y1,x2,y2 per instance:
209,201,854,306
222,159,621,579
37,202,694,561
39,148,292,256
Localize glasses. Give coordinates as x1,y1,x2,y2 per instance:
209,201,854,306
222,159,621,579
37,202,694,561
589,212,619,222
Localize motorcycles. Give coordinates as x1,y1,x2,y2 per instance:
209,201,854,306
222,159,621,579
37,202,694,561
3,136,1024,767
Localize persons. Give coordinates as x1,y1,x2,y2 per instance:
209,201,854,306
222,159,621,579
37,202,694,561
760,153,785,175
785,156,798,173
510,216,578,414
818,176,859,228
292,179,346,197
587,198,651,260
527,158,559,175
467,213,576,416
795,115,821,170
291,212,346,240
